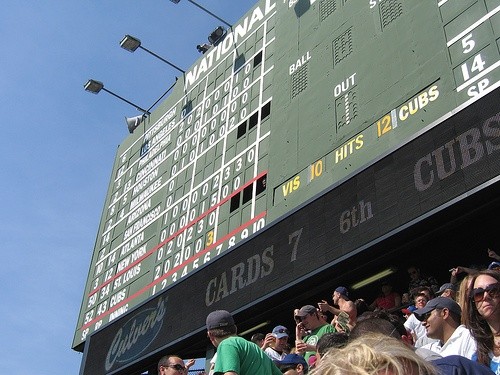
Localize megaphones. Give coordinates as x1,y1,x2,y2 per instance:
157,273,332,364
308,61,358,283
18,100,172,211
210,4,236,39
125,115,145,133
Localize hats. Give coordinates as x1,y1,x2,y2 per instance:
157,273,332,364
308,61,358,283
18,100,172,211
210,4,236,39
412,295,462,321
294,305,317,321
434,283,455,295
206,309,236,331
272,326,289,340
335,286,349,298
277,352,309,367
488,261,500,271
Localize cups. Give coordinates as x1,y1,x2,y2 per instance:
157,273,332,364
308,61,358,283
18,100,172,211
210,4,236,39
295,340,304,355
403,293,408,302
268,333,277,347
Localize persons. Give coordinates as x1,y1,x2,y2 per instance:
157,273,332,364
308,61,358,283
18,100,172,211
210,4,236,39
403,248,500,375
318,286,357,331
206,310,282,375
277,353,308,375
308,311,437,375
360,279,401,312
252,325,290,361
296,305,337,363
402,264,438,305
158,355,195,375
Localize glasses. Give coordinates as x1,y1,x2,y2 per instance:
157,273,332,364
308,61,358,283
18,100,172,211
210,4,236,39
163,364,185,371
275,329,290,334
407,271,417,276
469,283,500,303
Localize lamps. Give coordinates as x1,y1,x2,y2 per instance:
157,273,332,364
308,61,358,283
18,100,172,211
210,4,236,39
208,26,227,46
170,0,233,28
120,34,186,74
124,115,144,133
83,79,151,115
